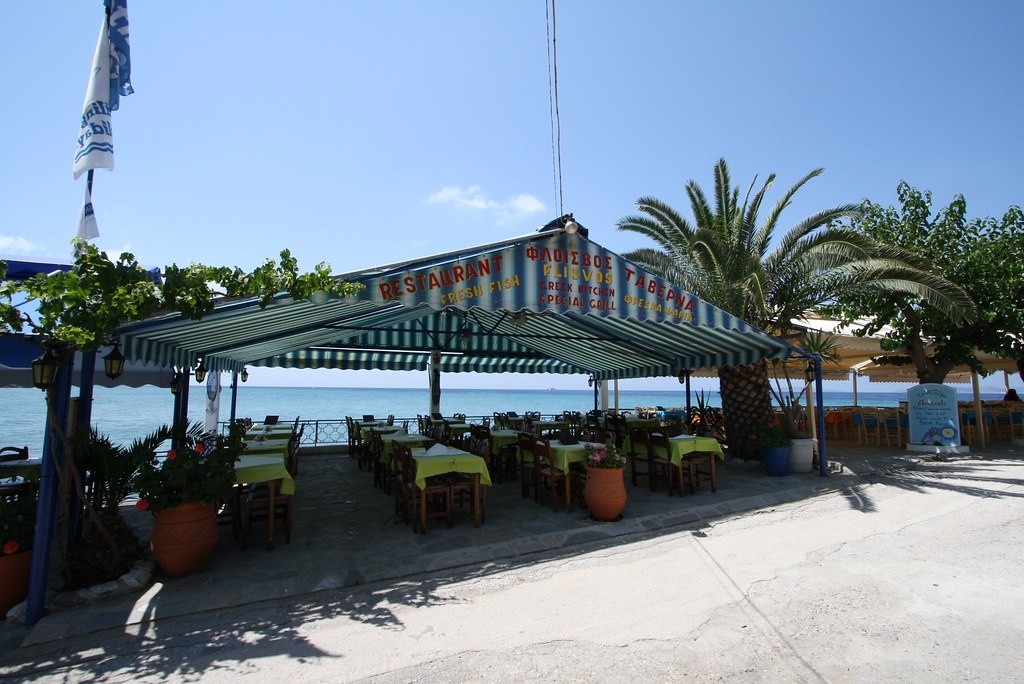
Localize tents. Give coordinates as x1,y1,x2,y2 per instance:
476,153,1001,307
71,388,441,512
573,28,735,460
0,230,1024,625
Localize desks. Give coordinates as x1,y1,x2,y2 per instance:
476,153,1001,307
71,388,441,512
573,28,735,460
240,438,290,458
251,421,294,432
772,401,1024,449
351,416,725,535
234,452,295,546
244,430,293,441
0,459,42,488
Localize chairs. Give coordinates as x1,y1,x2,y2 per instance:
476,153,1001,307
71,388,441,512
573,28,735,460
0,447,28,462
958,408,976,446
346,410,717,535
1008,406,1024,441
214,416,306,549
858,410,885,448
880,408,907,448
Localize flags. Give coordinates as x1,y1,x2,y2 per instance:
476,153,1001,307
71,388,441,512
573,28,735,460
73,0,134,179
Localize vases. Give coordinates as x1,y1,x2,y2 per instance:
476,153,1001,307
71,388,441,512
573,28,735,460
0,549,32,619
759,447,791,477
789,437,819,473
148,501,221,579
583,463,627,521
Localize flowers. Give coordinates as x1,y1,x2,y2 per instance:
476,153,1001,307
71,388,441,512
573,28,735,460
742,414,793,450
582,433,629,471
135,419,254,518
0,491,38,557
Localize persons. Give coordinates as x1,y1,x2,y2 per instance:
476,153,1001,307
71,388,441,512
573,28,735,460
1004,389,1023,423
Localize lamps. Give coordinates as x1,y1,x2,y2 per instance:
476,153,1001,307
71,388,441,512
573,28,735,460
101,344,128,381
589,376,594,387
30,343,62,392
805,361,817,383
169,378,181,395
195,360,208,384
678,371,686,383
241,368,249,382
